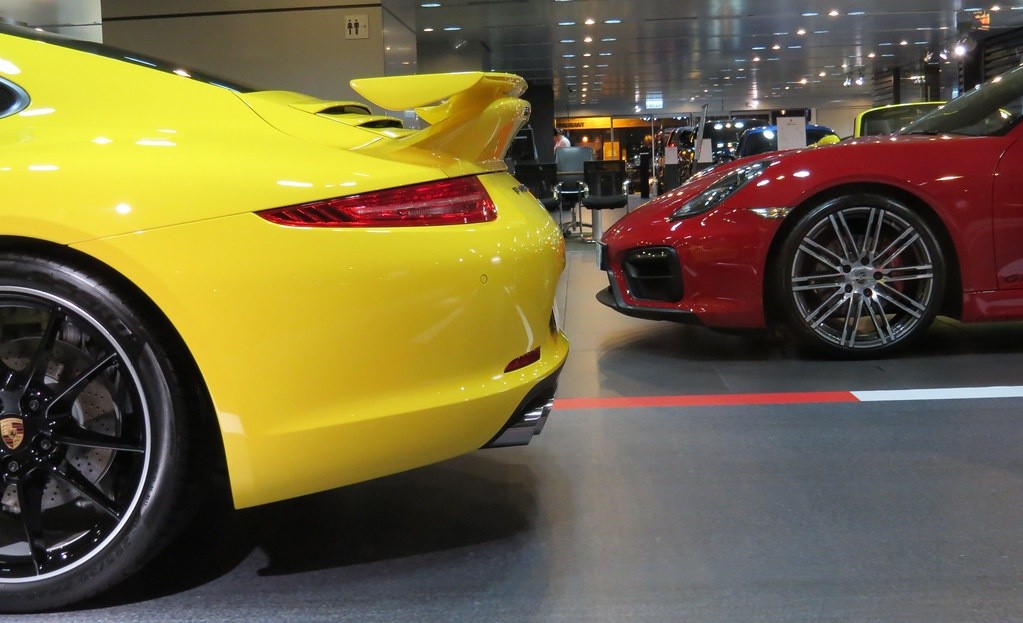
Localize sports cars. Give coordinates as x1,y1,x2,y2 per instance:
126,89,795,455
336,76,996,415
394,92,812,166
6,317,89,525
0,21,570,613
596,61,1023,361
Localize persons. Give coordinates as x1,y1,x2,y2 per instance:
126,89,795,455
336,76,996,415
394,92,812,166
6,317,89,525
553,128,574,162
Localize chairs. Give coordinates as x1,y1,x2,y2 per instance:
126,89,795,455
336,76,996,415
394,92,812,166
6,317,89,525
514,146,631,244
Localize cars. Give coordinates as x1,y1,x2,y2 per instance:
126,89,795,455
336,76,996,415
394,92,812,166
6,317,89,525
657,117,841,196
